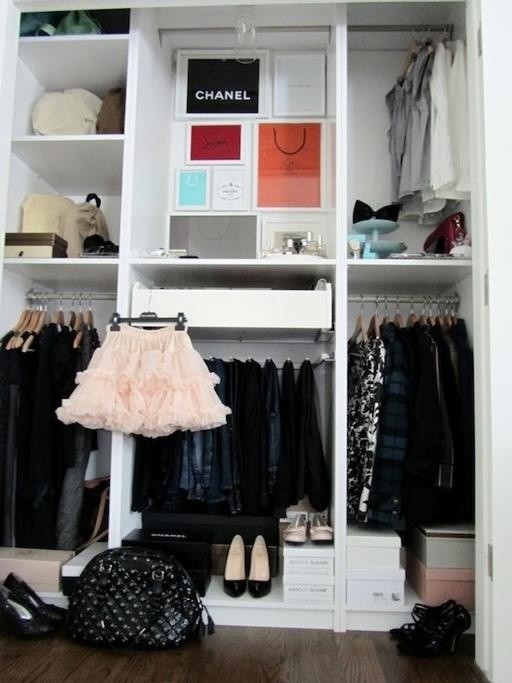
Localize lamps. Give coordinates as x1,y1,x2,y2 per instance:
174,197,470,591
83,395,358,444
231,5,260,64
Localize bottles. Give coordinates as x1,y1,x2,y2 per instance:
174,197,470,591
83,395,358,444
264,231,328,258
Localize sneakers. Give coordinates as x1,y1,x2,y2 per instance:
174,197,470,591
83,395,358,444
309,514,333,542
282,515,306,544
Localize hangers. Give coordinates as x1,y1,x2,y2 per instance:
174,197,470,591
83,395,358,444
6,286,99,352
109,288,189,333
399,21,462,84
349,290,460,343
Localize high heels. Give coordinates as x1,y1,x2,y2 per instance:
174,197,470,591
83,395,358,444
389,602,458,642
393,606,472,656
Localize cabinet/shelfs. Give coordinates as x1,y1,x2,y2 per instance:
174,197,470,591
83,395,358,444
1,1,512,682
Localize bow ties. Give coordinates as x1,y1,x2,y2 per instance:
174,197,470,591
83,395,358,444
353,200,402,221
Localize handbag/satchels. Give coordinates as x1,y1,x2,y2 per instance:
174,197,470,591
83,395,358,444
259,123,320,207
62,191,110,257
66,547,201,650
424,212,468,254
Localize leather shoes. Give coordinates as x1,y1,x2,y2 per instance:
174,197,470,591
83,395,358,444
249,533,270,597
1,570,66,625
224,535,246,598
0,588,64,640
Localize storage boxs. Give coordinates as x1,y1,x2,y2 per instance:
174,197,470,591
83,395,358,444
288,494,330,522
346,520,402,566
142,499,279,544
1,546,71,592
282,539,334,576
276,546,283,576
278,517,311,546
346,565,405,605
406,560,475,607
122,528,212,569
61,542,108,594
184,560,212,596
412,523,476,570
283,575,334,604
